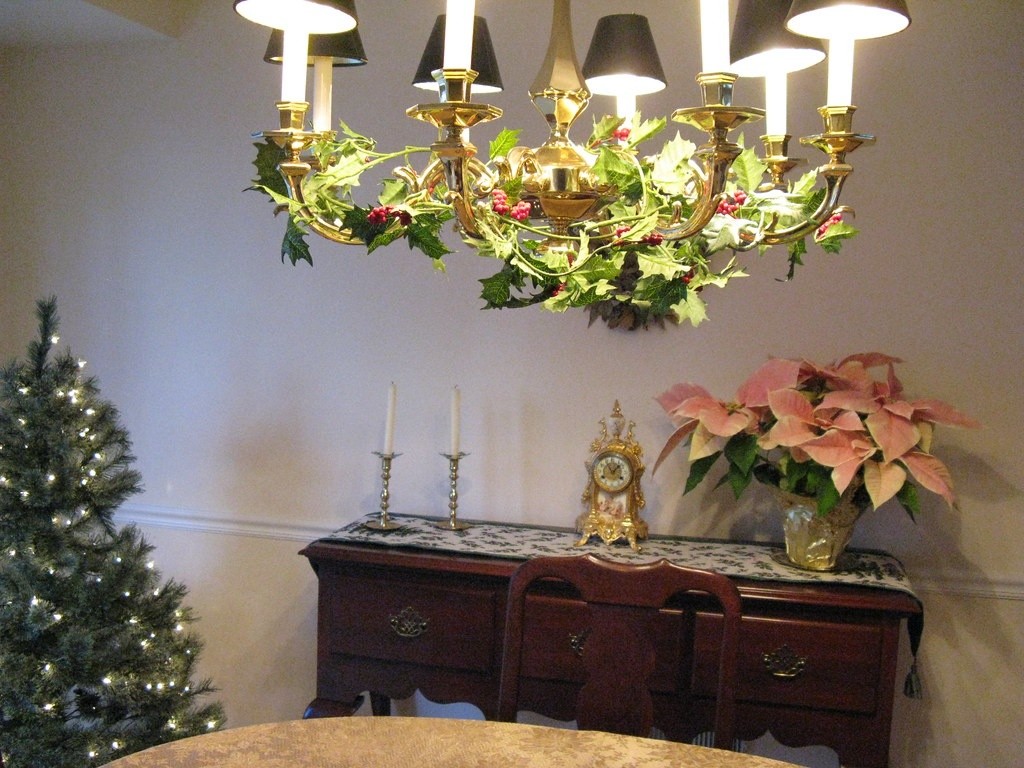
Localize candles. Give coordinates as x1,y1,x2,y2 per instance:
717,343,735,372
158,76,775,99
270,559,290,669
383,380,394,453
450,385,461,455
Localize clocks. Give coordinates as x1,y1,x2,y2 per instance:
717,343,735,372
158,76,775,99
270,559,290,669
577,398,649,552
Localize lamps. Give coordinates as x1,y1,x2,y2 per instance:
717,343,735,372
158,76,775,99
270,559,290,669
229,0,918,329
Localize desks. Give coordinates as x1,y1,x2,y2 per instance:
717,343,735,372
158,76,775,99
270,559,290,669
293,513,926,768
97,713,790,768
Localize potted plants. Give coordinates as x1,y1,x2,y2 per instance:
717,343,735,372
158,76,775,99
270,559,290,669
651,343,980,572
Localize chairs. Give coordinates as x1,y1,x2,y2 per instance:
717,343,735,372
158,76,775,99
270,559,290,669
494,553,742,751
